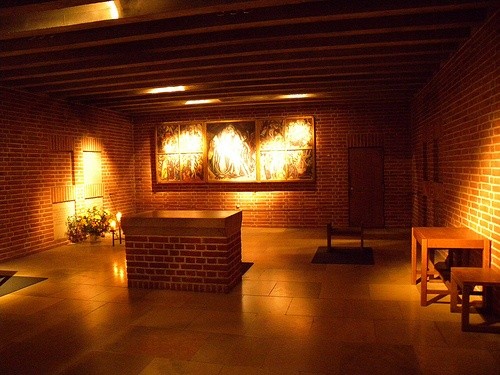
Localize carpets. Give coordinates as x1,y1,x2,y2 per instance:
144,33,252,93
311,245,374,265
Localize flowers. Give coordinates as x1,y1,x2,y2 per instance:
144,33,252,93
65,206,113,244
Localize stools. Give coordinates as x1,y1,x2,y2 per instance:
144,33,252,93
326,222,364,251
449,266,500,332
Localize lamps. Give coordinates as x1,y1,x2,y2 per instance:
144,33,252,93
116,211,124,240
110,220,116,246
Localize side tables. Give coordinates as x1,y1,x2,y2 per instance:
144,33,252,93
410,226,491,306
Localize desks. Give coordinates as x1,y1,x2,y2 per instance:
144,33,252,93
120,211,243,294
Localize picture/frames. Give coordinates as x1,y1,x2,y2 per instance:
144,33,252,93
154,115,317,185
